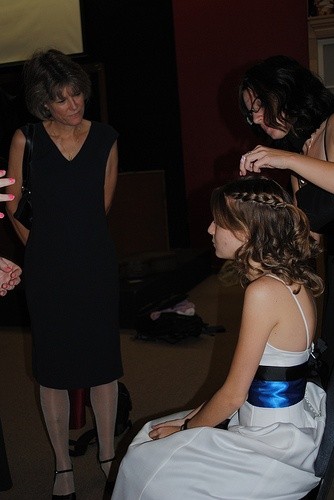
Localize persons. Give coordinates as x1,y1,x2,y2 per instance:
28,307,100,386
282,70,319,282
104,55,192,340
0,170,22,297
4,48,123,500
239,54,334,500
109,177,334,500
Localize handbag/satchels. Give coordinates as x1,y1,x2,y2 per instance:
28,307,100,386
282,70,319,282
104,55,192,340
133,290,225,346
13,124,35,231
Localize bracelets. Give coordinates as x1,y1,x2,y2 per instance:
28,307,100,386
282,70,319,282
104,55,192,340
182,418,190,430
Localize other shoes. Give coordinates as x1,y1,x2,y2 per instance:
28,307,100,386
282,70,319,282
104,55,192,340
52,470,77,500
99,458,114,479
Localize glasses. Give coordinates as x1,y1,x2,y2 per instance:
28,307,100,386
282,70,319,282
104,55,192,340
244,91,270,125
